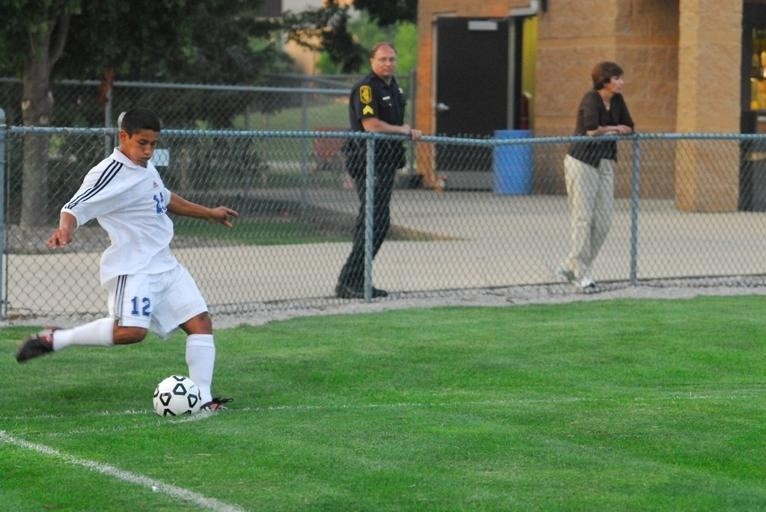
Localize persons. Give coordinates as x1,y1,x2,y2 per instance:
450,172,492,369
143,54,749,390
555,61,636,296
333,39,423,304
15,106,240,414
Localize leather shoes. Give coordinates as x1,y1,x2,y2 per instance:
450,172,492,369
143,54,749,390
335,278,387,299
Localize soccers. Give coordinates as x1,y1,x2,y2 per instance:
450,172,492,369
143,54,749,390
153,375,201,416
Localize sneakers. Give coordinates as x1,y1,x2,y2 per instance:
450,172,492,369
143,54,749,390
555,265,600,294
17,326,61,361
201,397,233,412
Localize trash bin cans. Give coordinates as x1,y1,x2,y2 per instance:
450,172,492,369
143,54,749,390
492,129,535,196
